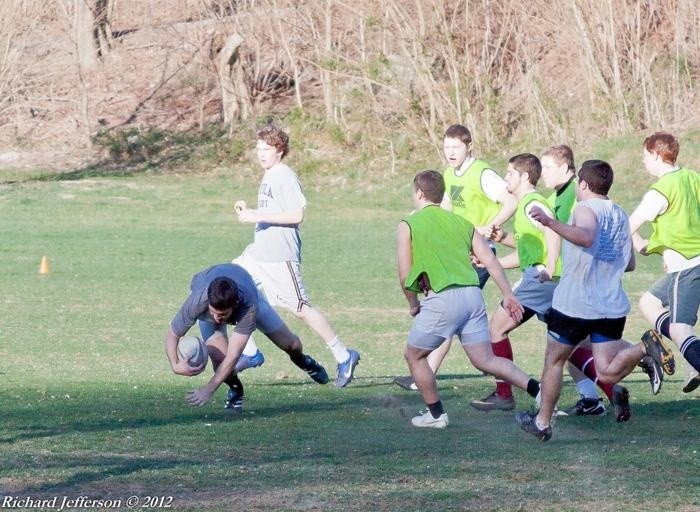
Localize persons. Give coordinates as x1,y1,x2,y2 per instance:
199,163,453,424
395,170,557,429
629,131,700,391
485,145,665,416
233,125,360,389
514,160,676,441
165,264,330,413
469,154,631,424
394,126,519,391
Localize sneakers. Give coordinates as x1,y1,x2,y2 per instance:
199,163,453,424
336,350,359,388
297,356,329,384
558,397,607,416
471,392,515,411
394,377,419,390
225,388,243,414
638,328,675,396
682,364,700,392
232,348,264,373
516,383,558,443
412,411,449,429
612,384,630,422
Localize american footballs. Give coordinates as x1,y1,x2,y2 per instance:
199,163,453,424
177,335,207,367
